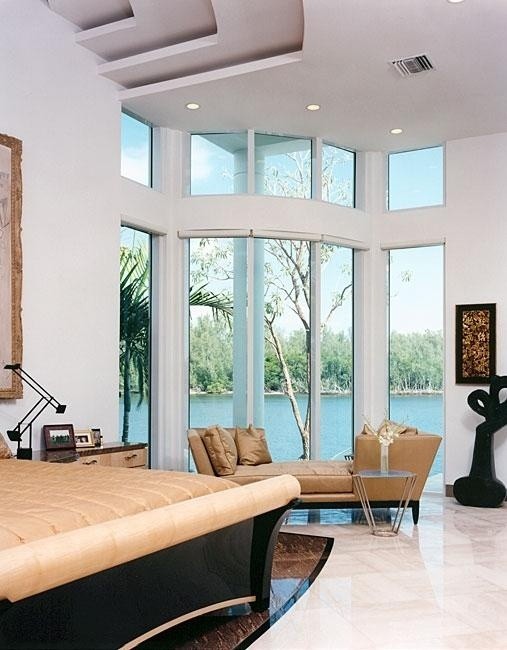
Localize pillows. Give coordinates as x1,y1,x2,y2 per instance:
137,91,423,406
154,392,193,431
347,422,418,474
200,425,237,476
235,424,272,466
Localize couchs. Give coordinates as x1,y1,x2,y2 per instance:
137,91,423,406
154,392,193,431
187,420,442,525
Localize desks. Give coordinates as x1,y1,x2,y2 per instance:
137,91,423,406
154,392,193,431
353,470,419,537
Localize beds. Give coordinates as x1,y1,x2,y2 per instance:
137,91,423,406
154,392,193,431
0,432,304,650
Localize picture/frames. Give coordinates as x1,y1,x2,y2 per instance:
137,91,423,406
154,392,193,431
44,424,76,451
455,303,496,384
92,429,100,446
74,429,95,448
0,133,23,399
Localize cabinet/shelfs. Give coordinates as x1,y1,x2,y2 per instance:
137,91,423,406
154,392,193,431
76,442,148,470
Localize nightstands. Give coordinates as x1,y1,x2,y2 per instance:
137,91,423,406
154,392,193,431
14,450,79,465
